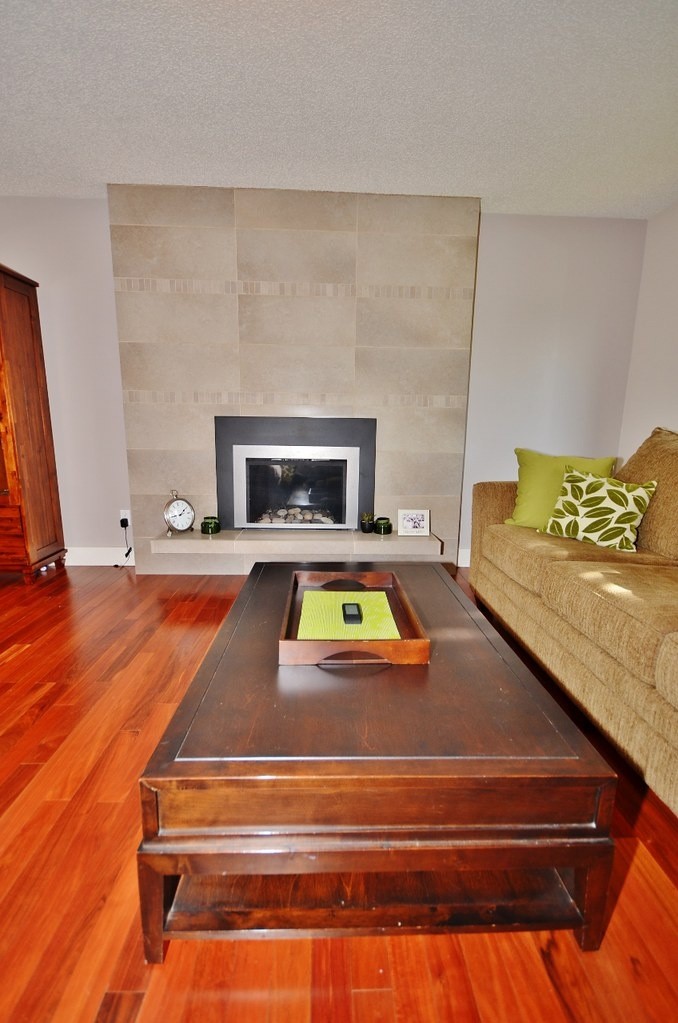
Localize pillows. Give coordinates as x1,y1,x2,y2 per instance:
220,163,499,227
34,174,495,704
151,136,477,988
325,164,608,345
536,464,658,553
503,447,616,527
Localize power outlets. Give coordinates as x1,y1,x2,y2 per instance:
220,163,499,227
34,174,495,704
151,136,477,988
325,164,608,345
120,510,131,527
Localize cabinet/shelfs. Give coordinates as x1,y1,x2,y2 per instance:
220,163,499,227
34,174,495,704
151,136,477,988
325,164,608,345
0,263,67,587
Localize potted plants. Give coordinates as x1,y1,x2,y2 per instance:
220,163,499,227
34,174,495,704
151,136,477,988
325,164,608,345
360,512,375,533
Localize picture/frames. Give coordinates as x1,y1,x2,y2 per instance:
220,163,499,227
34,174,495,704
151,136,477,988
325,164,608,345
398,509,430,538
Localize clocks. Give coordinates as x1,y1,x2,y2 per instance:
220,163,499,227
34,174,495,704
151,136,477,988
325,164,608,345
163,490,196,538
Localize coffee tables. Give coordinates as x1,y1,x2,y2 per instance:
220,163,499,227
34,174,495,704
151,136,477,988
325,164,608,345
135,560,615,964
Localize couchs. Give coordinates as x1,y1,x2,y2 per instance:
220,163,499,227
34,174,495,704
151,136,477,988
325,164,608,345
472,425,678,819
